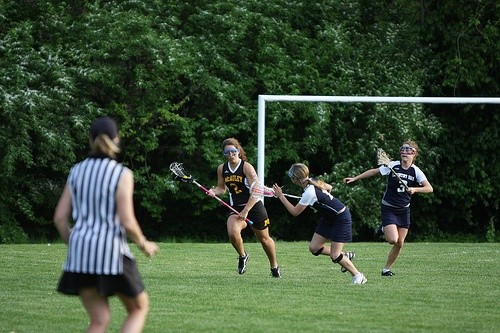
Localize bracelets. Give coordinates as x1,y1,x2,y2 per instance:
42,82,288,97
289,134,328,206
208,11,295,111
142,241,148,251
244,208,249,212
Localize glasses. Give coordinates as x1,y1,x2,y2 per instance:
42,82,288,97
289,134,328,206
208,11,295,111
223,148,238,156
399,145,416,152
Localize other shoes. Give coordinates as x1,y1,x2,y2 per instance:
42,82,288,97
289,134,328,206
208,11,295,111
381,267,395,277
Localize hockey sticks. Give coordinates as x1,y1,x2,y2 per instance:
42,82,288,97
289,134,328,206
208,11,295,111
250,179,303,201
170,161,255,226
376,145,411,190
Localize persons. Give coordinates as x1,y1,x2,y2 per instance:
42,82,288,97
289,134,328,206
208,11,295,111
343,141,433,276
205,138,281,277
272,163,367,284
54,117,159,333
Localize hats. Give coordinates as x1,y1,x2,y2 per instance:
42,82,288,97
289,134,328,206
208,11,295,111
91,117,117,142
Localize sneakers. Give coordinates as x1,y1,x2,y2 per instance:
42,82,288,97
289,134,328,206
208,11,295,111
352,272,367,285
340,251,355,272
270,265,280,277
237,250,250,274
